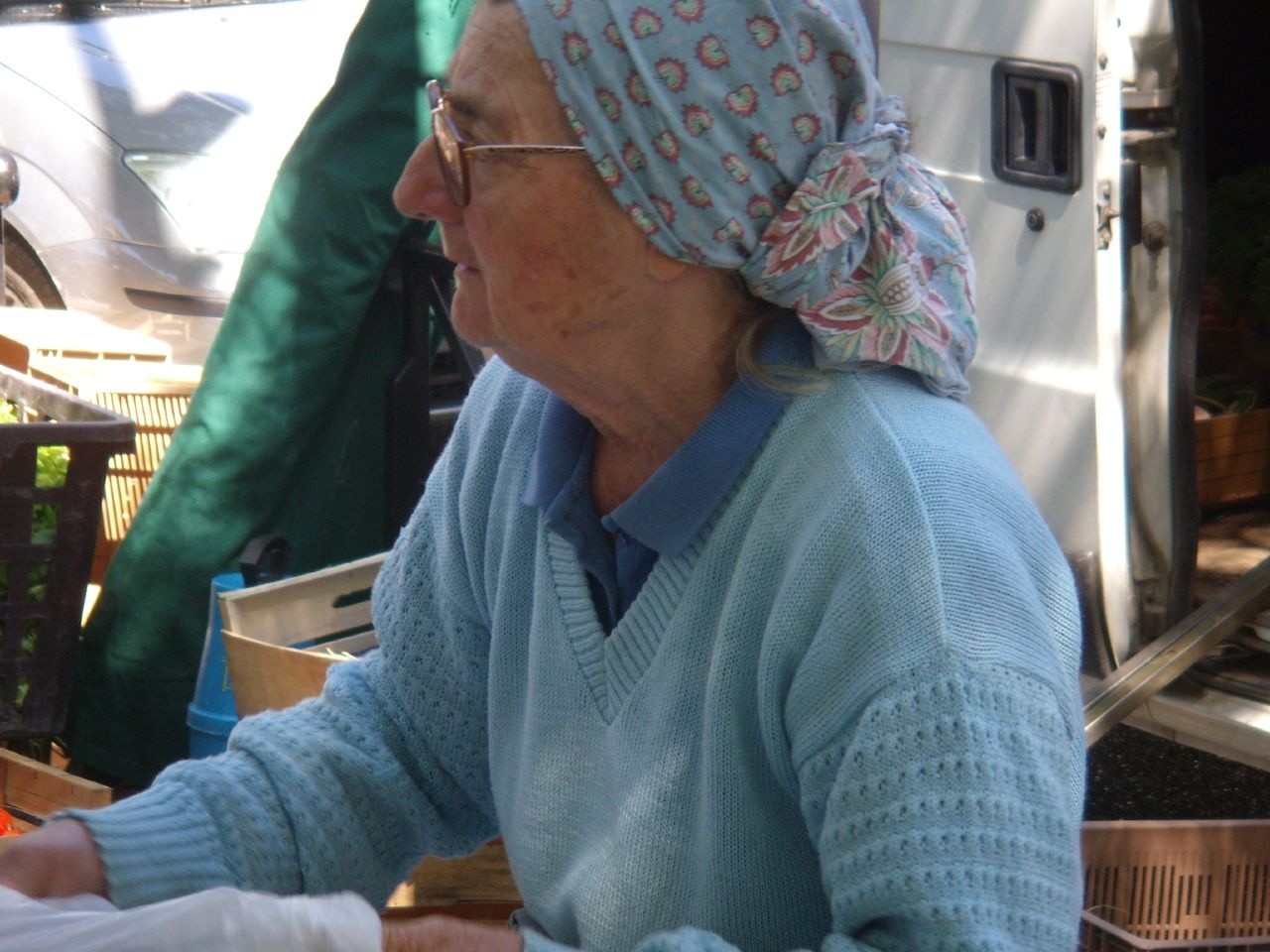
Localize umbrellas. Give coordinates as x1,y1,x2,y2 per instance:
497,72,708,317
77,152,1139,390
26,0,475,801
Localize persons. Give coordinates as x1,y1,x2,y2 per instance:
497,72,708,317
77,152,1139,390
0,0,1087,952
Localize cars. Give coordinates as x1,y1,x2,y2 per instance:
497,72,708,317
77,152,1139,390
0,1,374,411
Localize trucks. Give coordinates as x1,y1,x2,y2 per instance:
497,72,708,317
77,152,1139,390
856,1,1270,782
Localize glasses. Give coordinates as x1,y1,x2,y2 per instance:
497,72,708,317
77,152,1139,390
427,78,588,207
